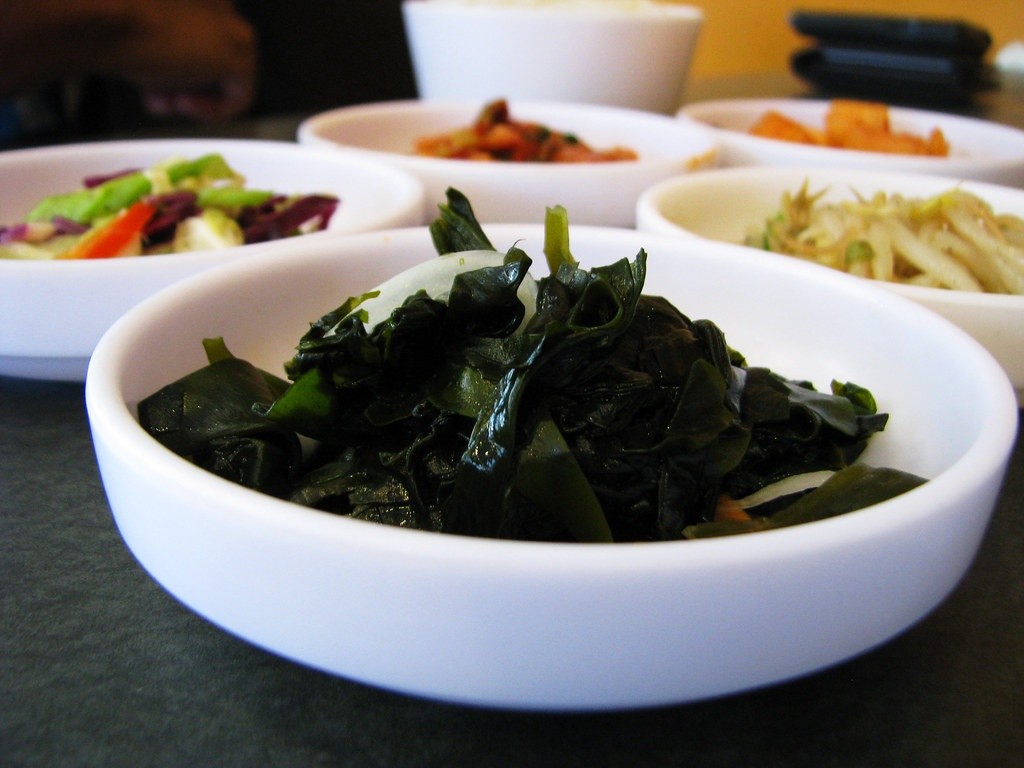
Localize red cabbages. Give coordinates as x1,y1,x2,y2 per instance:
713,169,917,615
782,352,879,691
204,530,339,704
0,167,340,247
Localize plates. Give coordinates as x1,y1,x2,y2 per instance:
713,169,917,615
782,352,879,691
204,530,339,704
676,95,1024,186
296,95,719,232
0,135,423,387
84,218,1024,711
634,168,1024,389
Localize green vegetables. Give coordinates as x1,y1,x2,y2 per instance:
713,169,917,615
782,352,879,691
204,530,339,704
136,181,929,543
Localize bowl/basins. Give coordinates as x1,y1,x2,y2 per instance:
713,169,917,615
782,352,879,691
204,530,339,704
403,0,704,119
